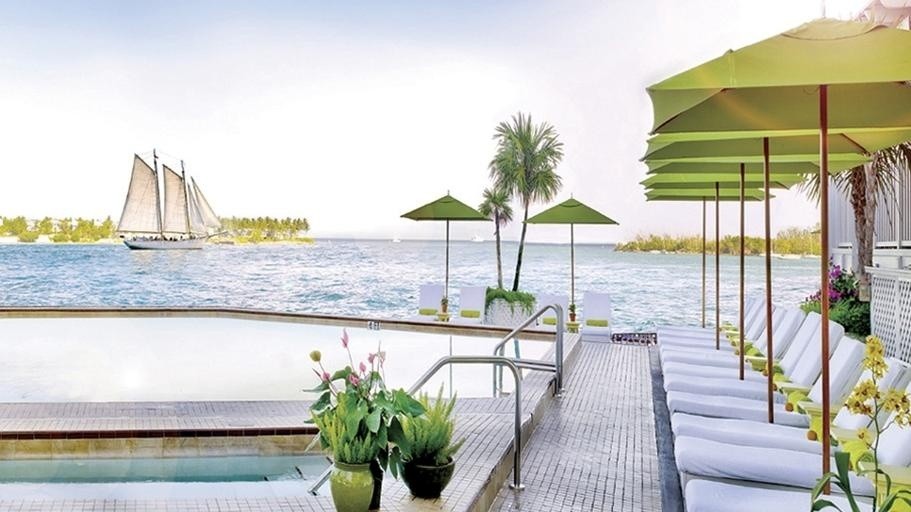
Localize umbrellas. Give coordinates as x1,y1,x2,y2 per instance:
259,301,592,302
399,189,496,301
522,190,622,317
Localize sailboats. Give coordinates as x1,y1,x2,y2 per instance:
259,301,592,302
116,147,229,250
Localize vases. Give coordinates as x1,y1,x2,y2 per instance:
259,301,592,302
330,463,374,509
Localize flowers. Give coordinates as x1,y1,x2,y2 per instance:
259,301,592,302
307,329,427,462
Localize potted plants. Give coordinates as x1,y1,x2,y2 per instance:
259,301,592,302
396,381,467,498
479,110,565,328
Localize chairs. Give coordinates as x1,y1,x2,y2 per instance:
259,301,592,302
671,356,910,455
666,335,874,426
536,292,614,340
685,479,883,510
658,295,844,405
674,380,911,498
408,283,488,326
